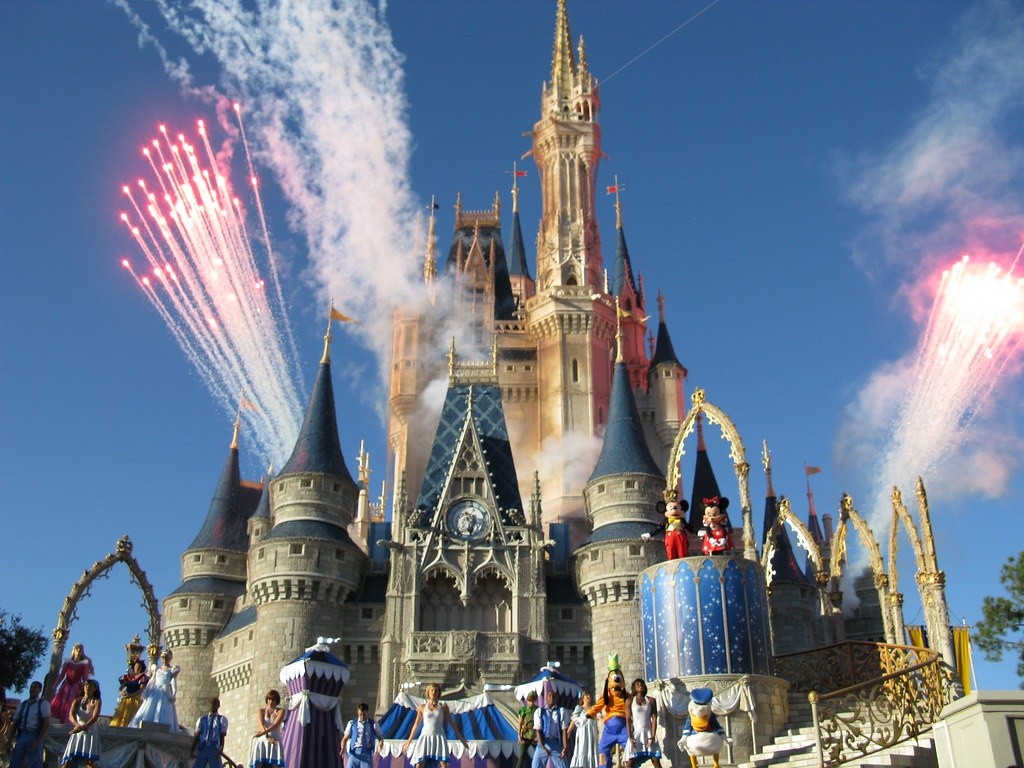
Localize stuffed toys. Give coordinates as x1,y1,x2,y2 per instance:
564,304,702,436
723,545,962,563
677,688,734,768
699,497,736,556
641,500,705,560
574,667,635,768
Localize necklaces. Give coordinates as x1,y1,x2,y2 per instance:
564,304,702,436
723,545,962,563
432,705,435,707
82,700,88,704
268,710,273,713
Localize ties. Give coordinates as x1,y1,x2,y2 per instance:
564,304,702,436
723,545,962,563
23,701,37,736
545,709,555,738
206,714,216,745
359,722,368,750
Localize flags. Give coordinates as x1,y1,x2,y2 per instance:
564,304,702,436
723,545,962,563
331,308,365,329
807,466,821,474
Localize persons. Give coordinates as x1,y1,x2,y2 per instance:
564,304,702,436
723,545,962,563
110,660,151,727
187,697,229,768
248,690,285,768
340,703,383,768
567,690,604,768
51,643,94,724
516,691,540,768
61,679,102,768
4,681,51,768
128,650,181,734
622,679,663,768
532,691,569,768
403,684,469,768
